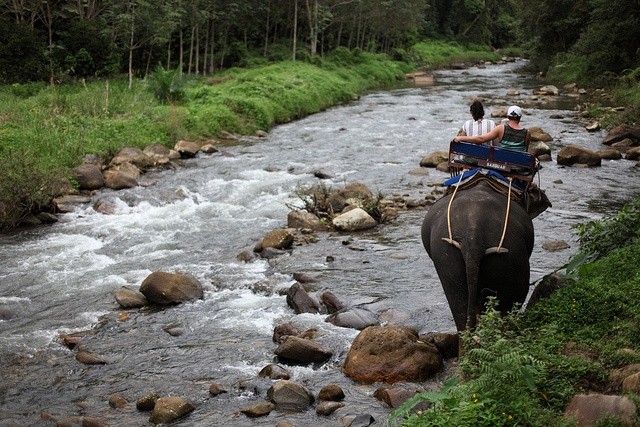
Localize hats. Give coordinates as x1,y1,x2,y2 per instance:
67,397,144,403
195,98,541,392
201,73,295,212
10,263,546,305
507,105,522,117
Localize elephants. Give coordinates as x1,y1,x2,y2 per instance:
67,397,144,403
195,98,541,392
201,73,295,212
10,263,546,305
420,176,553,334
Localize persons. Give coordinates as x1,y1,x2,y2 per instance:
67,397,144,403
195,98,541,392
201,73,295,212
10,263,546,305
458,101,499,147
451,105,532,172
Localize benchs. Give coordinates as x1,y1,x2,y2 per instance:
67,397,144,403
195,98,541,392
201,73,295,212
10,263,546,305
447,140,540,193
